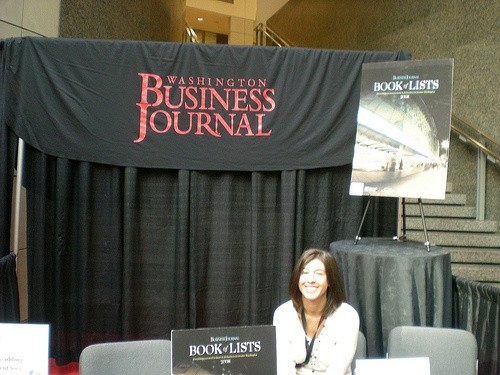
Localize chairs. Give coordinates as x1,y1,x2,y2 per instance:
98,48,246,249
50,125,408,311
386,327,478,375
78,340,171,375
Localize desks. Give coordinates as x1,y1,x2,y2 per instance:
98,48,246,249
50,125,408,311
331,238,453,360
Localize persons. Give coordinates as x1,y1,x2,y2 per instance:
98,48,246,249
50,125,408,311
272,247,361,375
